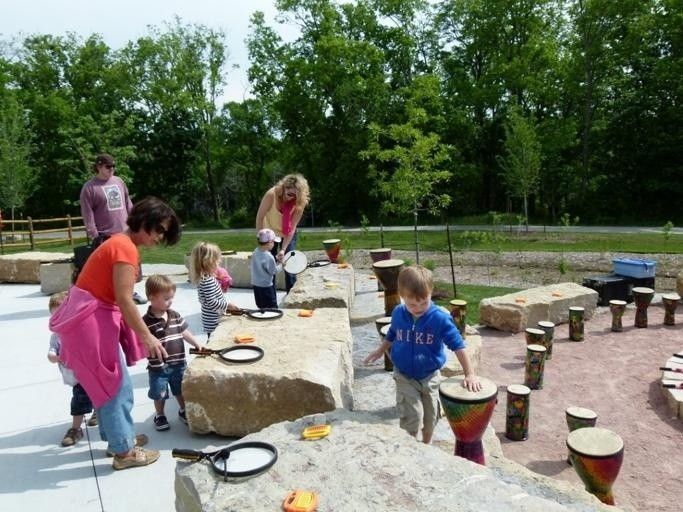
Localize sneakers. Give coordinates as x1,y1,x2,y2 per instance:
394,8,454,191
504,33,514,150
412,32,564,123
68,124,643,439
88,409,97,426
106,434,160,470
152,415,170,431
62,427,84,446
132,292,147,304
178,410,188,424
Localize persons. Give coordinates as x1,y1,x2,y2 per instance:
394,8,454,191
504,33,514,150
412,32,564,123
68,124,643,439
189,241,241,343
255,172,310,300
48,194,182,470
249,228,287,310
45,288,101,448
80,152,149,306
139,273,209,431
362,265,484,446
213,264,233,292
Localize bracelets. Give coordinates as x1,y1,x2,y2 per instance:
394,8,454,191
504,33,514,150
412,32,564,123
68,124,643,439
281,249,286,252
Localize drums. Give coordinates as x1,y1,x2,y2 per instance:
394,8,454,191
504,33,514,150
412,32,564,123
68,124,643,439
372,258,404,317
375,316,393,372
506,287,683,506
321,237,342,263
379,322,392,362
449,299,467,341
368,246,392,293
439,374,498,467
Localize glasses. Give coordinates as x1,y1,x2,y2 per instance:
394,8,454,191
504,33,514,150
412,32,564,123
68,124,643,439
106,164,115,169
155,224,168,238
287,188,296,197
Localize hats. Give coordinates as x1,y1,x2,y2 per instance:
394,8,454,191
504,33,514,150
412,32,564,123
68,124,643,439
256,228,282,243
96,155,113,165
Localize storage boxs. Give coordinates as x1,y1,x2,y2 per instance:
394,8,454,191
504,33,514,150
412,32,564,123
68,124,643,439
611,258,656,278
611,275,655,303
582,275,627,306
